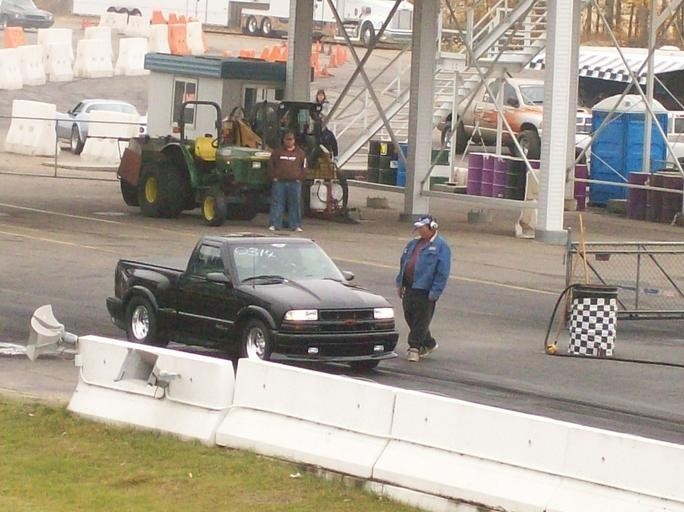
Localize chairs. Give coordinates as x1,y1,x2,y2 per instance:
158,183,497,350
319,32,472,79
194,137,218,162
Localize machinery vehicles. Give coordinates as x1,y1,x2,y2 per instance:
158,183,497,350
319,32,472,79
112,91,336,227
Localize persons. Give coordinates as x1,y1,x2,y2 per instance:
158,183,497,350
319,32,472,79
311,89,331,131
266,132,307,233
394,215,451,362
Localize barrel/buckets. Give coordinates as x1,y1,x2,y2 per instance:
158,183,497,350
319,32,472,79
626,167,684,227
365,138,451,189
465,151,588,210
566,283,620,357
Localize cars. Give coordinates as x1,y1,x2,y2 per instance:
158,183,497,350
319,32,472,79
0,0,57,32
52,97,146,152
660,108,684,174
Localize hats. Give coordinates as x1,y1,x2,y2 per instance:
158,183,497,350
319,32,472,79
285,132,294,140
413,216,438,228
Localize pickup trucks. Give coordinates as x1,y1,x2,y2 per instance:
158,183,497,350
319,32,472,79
436,77,593,162
101,226,402,377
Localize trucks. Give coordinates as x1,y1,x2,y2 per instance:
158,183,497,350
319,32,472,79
71,0,415,53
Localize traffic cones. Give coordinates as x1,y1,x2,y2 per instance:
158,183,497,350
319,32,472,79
223,40,349,79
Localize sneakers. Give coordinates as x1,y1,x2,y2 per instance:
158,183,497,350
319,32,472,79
420,343,439,358
407,347,420,362
294,226,302,233
268,225,275,231
